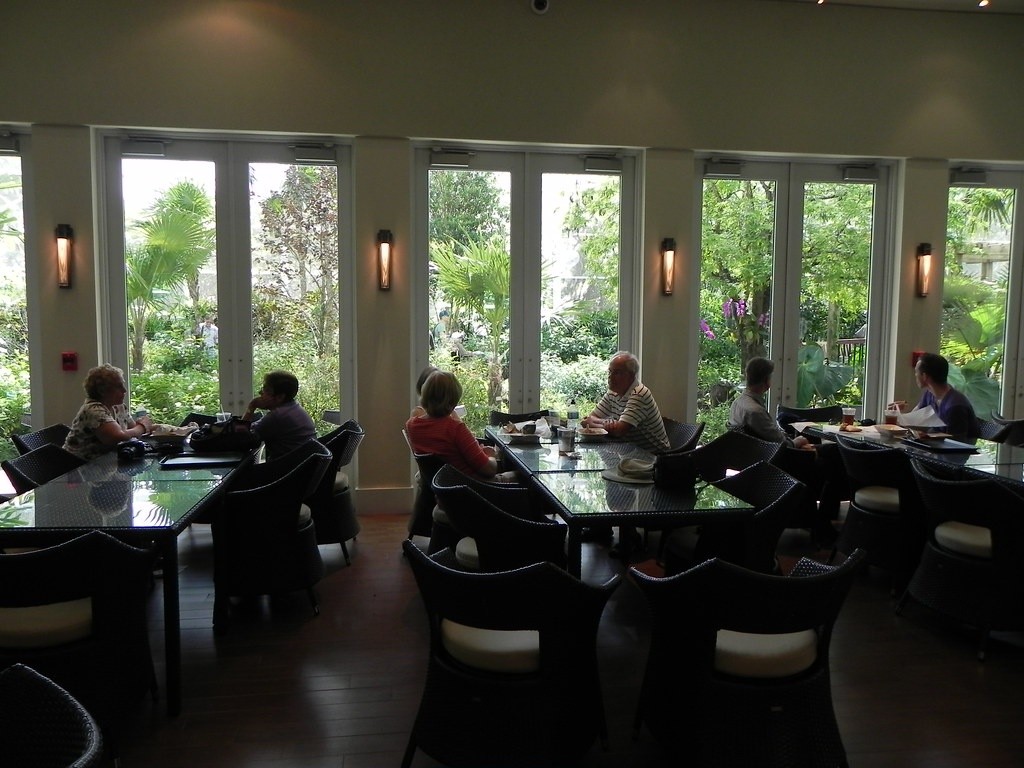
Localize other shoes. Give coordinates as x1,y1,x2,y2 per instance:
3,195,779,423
609,535,641,560
581,529,612,541
811,518,841,542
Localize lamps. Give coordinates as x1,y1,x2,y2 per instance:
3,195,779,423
661,238,676,295
55,224,73,288
378,229,393,289
917,243,931,297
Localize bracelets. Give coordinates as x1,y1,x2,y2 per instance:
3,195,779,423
138,423,146,434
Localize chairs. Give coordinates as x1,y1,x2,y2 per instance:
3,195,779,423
0,404,1024,768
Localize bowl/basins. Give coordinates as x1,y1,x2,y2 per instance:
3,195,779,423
149,428,193,443
510,434,540,443
578,428,609,440
875,424,908,438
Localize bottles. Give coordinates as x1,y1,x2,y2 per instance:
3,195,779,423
568,400,579,428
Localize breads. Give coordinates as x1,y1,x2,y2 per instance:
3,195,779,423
839,423,863,432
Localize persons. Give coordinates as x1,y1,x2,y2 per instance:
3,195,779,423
435,311,449,339
729,358,842,516
235,372,317,462
62,363,153,460
199,315,218,358
78,453,130,518
889,353,981,438
594,442,659,511
406,367,522,483
581,351,671,542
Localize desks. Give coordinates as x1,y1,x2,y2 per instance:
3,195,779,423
965,465,1024,490
486,424,631,452
794,422,910,449
505,445,658,480
111,437,265,458
0,480,223,718
866,438,1024,473
531,472,755,581
48,454,246,627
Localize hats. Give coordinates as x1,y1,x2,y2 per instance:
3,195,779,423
602,458,655,484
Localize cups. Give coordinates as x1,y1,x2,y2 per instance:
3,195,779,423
884,410,899,425
216,413,232,423
842,407,856,425
556,427,575,455
547,417,560,426
523,424,536,434
